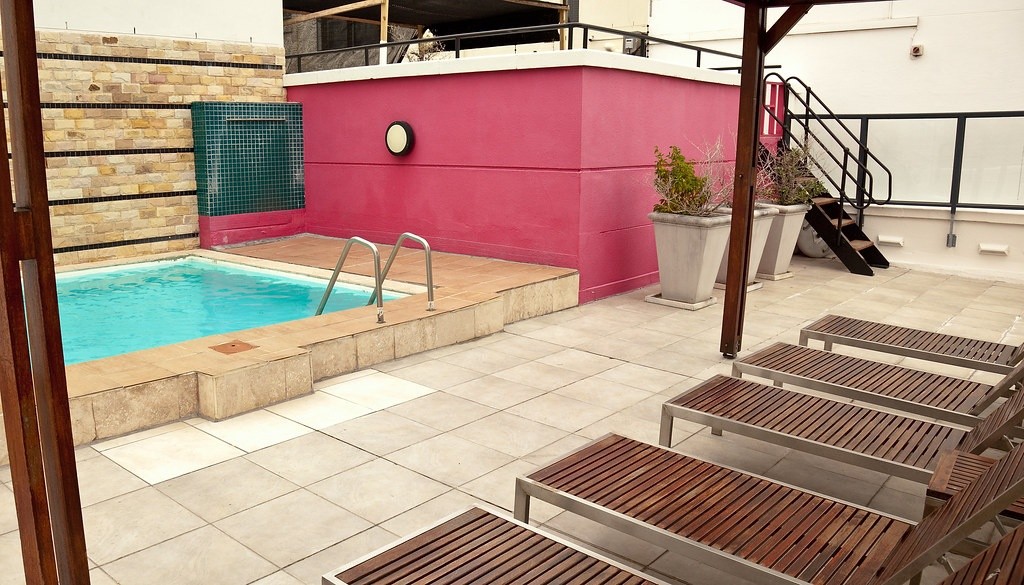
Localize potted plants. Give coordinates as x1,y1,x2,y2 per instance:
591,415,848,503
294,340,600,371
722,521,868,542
647,140,830,304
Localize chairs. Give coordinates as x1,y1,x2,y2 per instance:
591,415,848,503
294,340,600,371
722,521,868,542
322,312,1023,585
